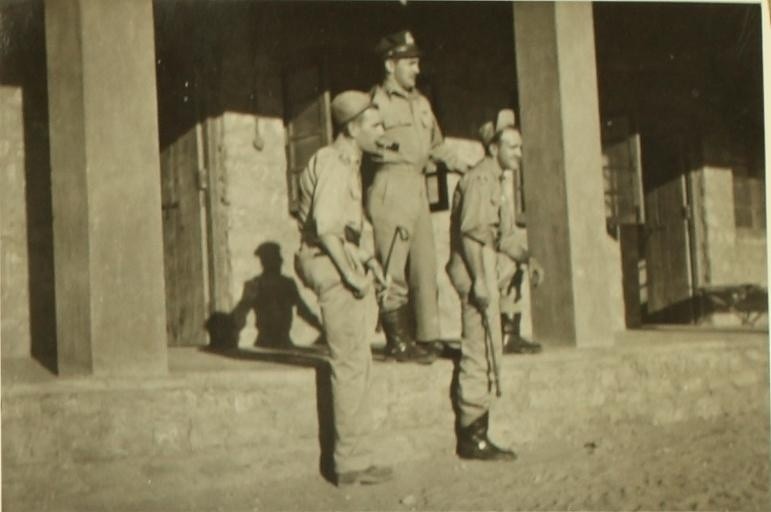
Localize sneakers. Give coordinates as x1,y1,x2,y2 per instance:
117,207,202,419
337,467,393,487
459,430,517,461
506,335,543,353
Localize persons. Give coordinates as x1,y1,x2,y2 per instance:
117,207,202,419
444,106,547,461
364,29,474,363
291,89,392,487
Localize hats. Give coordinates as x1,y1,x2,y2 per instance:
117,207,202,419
375,31,424,59
333,89,373,124
477,108,519,143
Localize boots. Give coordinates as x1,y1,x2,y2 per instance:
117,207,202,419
381,307,433,365
